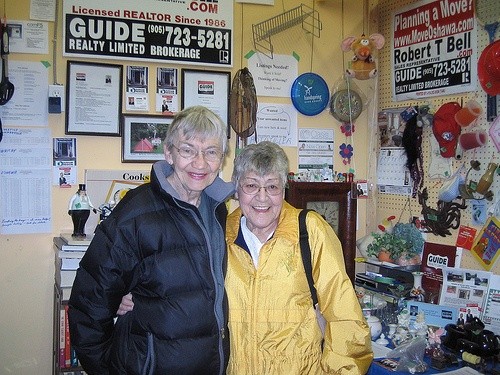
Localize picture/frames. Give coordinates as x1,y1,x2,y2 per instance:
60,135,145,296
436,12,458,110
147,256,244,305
121,113,176,164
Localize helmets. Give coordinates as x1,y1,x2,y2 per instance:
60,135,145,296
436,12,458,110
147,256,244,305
478,40,500,97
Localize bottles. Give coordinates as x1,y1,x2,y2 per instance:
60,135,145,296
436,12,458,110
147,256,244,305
477,163,497,195
410,272,425,302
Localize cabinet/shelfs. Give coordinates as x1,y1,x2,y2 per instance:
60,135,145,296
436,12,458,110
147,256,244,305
285,180,357,293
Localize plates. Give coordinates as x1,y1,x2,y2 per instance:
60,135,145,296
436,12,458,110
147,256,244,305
291,72,330,116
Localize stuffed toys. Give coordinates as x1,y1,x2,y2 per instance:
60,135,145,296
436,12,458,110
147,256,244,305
341,33,386,80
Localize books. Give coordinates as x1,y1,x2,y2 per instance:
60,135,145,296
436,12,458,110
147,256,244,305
53,237,89,369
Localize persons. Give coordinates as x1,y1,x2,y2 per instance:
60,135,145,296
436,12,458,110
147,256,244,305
67,106,237,375
466,309,473,323
59,173,66,186
68,184,97,237
457,313,465,326
117,141,374,375
162,100,169,112
410,306,418,316
149,130,159,139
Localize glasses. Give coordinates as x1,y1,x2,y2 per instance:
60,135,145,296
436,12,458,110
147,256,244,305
171,143,223,160
238,179,285,195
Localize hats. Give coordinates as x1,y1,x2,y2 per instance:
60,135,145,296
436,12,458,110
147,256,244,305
432,102,461,158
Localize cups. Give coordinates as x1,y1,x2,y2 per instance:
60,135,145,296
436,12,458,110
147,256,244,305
365,314,383,341
459,130,487,151
455,101,483,126
